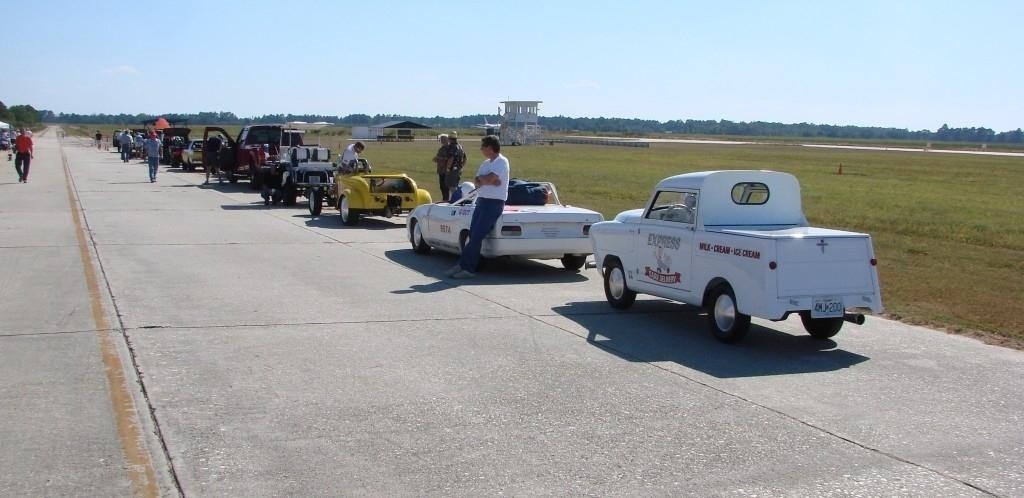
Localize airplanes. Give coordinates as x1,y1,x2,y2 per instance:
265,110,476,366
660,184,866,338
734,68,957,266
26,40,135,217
472,116,501,129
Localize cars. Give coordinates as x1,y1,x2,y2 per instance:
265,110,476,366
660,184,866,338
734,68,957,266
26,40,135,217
113,125,192,167
182,138,209,172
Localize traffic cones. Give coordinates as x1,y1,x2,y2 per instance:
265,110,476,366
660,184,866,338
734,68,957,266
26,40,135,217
837,163,844,175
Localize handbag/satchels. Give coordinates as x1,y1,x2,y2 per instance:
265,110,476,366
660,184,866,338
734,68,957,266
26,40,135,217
505,178,550,205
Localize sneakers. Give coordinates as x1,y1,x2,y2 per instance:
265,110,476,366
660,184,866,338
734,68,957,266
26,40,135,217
446,265,475,278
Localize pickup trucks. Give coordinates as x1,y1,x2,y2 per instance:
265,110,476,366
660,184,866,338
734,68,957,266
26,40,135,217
203,124,322,188
587,166,883,345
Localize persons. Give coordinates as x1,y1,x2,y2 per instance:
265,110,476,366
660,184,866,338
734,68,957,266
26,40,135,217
342,141,364,173
203,134,224,185
15,128,33,183
116,128,159,164
432,133,448,200
94,129,103,151
144,131,164,182
446,130,467,201
445,134,510,278
1,128,33,156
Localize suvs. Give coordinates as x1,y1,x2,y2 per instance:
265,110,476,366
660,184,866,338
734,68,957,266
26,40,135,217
260,121,344,209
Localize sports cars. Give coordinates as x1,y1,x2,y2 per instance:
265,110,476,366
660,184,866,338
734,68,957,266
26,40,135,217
308,158,432,228
407,182,604,272
